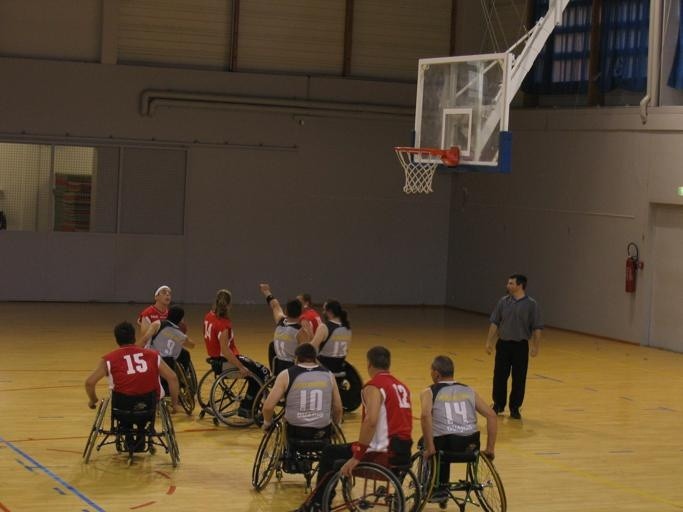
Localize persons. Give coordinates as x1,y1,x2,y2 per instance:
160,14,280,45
317,345,411,511
260,284,309,375
262,343,344,472
410,355,499,508
295,293,324,342
136,285,189,368
85,320,179,451
135,305,195,393
203,290,273,427
310,300,352,370
486,273,544,419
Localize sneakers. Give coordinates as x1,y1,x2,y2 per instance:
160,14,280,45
509,407,523,419
492,405,504,414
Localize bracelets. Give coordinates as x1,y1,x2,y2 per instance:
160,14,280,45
352,444,367,461
266,294,275,303
264,420,272,427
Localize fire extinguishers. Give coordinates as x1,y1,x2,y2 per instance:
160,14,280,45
625,242,639,293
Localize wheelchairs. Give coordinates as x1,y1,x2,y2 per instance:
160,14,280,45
83,397,180,467
320,448,507,512
175,360,362,428
252,422,356,491
